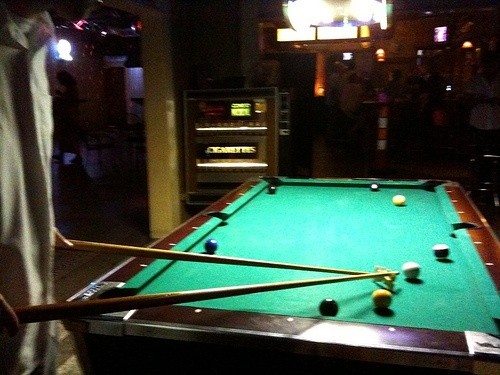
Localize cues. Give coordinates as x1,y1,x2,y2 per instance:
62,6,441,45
9,271,400,324
52,237,387,278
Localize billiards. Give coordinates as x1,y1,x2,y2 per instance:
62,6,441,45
205,238,219,254
392,195,405,205
372,289,393,308
269,184,278,194
432,244,450,260
402,263,420,279
319,298,337,317
369,182,379,191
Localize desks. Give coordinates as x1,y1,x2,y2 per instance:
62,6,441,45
64,173,499,375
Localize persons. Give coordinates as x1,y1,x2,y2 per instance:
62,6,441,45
53,70,84,164
317,52,499,181
0,0,73,373
